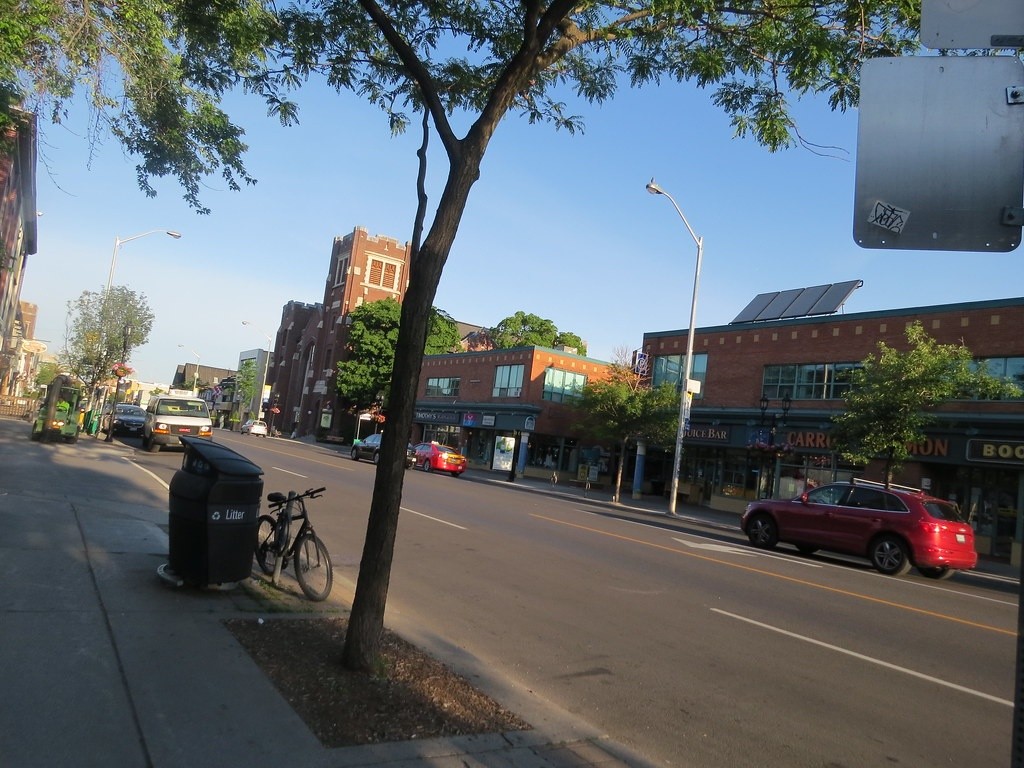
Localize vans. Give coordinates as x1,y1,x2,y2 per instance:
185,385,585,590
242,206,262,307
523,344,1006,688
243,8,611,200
145,395,216,454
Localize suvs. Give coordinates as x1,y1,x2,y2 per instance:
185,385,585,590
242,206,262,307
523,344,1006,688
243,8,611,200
351,432,416,470
740,477,978,582
240,419,267,438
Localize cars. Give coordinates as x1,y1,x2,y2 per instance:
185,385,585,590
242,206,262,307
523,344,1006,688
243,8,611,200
103,404,148,439
414,441,466,478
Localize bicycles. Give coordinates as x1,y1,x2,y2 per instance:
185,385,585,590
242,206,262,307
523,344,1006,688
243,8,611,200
256,486,333,603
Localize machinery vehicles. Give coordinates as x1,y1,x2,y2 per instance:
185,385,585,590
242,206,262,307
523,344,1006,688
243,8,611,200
31,373,87,444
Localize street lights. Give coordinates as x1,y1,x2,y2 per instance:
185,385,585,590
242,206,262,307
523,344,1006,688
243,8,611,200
103,322,134,442
241,320,272,424
644,176,705,517
177,343,201,396
86,229,182,413
